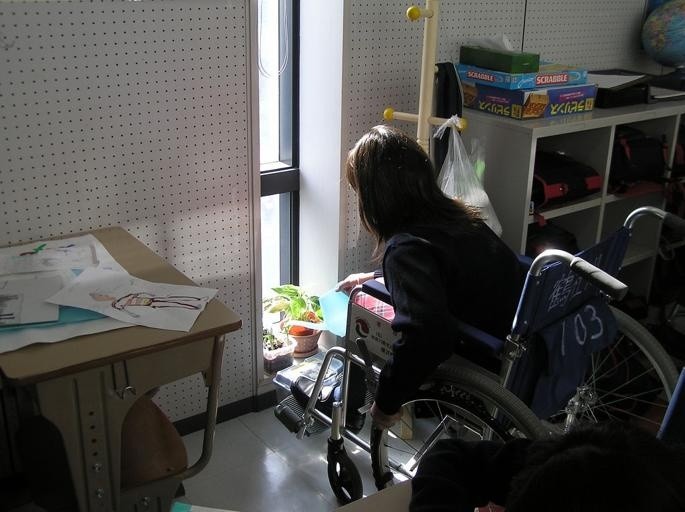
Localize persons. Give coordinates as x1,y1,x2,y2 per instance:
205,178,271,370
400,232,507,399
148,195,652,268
289,124,529,436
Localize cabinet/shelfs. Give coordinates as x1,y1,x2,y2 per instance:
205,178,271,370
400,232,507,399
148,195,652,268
461,101,685,307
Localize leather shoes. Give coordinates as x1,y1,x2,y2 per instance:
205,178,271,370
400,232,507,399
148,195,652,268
291,375,364,434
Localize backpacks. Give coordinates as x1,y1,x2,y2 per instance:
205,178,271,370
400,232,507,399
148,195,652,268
530,150,602,209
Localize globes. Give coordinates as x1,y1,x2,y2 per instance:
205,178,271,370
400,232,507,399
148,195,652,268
643,0,685,78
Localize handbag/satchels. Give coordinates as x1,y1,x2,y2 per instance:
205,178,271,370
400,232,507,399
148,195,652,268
616,125,672,187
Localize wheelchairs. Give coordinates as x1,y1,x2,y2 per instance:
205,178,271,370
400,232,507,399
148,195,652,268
270,204,685,506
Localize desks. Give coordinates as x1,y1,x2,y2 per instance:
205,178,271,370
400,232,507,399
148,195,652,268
2,225,247,511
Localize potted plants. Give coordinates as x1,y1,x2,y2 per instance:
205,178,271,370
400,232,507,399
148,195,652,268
262,285,323,369
